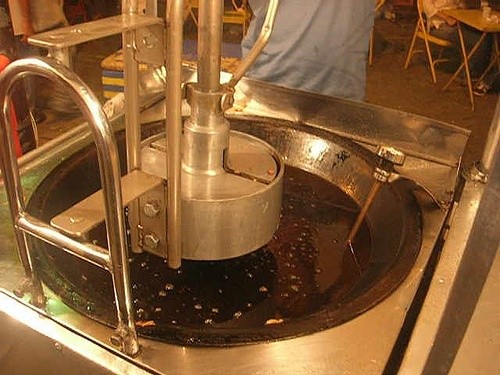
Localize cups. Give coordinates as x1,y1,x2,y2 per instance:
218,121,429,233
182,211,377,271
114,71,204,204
482,7,492,21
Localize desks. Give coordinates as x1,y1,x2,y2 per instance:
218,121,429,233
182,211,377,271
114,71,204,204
442,9,500,112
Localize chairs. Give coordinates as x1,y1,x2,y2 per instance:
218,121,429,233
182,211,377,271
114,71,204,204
405,0,459,84
367,0,386,65
222,11,249,39
184,0,199,28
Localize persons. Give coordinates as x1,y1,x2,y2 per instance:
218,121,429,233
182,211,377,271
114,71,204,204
241,0,377,103
7,0,66,124
423,1,493,91
